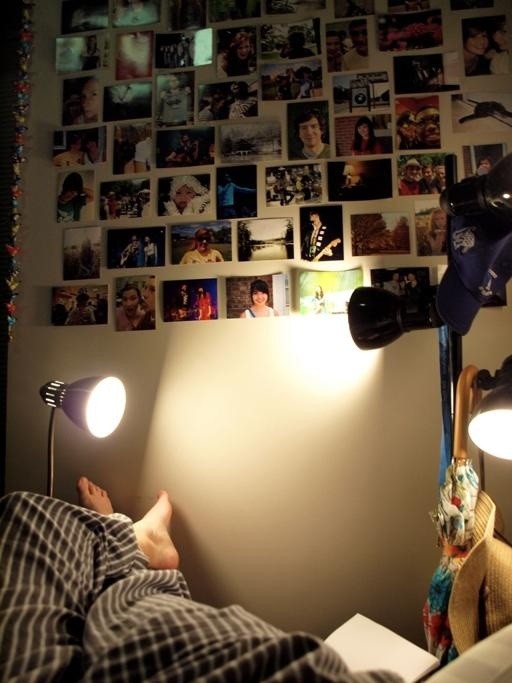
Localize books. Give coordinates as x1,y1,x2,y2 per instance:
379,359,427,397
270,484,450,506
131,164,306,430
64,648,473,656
323,611,441,683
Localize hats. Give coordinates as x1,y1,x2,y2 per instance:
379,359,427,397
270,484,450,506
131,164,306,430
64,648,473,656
286,24,305,39
435,204,511,336
405,158,420,167
447,488,512,655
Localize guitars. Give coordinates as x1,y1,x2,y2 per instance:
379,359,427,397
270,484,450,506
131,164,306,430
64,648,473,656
312,238,340,261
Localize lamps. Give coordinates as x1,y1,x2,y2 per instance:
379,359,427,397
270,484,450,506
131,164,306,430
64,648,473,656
465,355,512,463
38,374,126,501
347,282,465,466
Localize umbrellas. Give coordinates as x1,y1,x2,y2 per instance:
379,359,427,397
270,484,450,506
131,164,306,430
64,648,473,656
421,362,484,665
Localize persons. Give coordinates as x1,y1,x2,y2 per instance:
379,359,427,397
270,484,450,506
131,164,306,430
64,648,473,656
50,1,508,331
0,476,408,683
